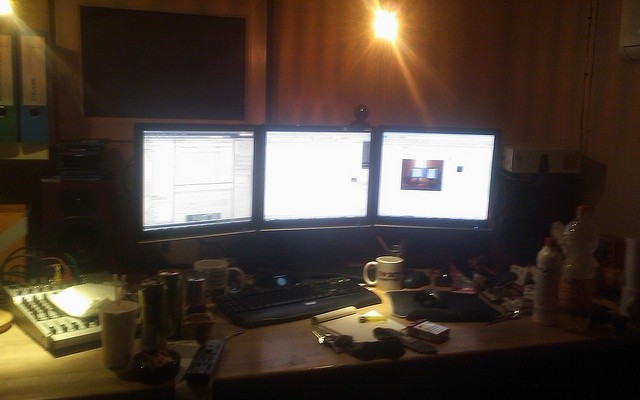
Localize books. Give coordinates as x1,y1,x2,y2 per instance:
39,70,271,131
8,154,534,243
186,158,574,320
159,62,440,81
594,233,639,305
311,307,404,342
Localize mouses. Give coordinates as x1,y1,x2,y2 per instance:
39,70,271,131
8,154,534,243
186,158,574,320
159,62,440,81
414,288,448,308
403,272,430,287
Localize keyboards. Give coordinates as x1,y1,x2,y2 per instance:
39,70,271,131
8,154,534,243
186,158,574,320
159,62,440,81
215,276,383,330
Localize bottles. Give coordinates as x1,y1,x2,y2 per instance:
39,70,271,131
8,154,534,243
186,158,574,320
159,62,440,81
532,236,562,326
556,205,600,315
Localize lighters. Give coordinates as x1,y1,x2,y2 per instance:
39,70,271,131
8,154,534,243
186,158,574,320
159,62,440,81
359,314,387,323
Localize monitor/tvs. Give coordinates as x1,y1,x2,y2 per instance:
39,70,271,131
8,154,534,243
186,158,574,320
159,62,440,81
262,125,374,233
133,126,259,244
375,127,500,232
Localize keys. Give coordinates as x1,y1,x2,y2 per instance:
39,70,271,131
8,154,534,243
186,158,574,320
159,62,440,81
311,329,326,344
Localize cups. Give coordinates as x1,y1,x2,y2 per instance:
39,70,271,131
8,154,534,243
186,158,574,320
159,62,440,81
193,259,245,303
363,256,404,290
98,301,139,368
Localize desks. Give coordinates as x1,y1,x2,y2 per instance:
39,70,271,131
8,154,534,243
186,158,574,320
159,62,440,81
1,268,640,399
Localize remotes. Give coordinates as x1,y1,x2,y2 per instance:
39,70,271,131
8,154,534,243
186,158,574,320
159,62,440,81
187,338,224,381
